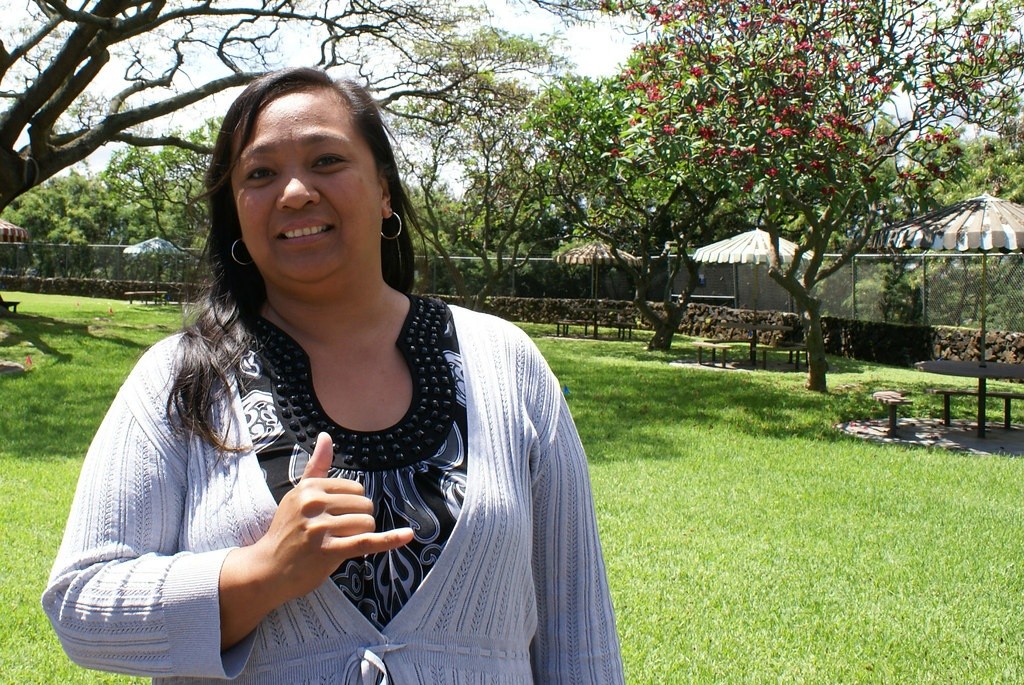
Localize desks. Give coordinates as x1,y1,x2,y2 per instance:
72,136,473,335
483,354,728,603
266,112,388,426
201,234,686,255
915,360,1024,439
575,307,618,338
724,322,793,367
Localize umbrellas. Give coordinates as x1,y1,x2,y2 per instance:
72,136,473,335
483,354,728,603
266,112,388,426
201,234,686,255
552,241,643,309
692,227,825,326
869,192,1024,367
0,219,29,244
121,236,186,291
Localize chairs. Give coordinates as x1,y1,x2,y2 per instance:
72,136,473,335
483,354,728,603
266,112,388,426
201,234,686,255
0,295,20,312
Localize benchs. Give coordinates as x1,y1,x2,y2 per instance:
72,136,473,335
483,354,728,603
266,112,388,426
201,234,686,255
691,339,808,371
124,291,167,304
933,389,1024,429
554,320,635,340
872,390,912,437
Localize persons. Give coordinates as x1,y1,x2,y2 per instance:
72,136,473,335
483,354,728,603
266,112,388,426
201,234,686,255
40,69,626,685
